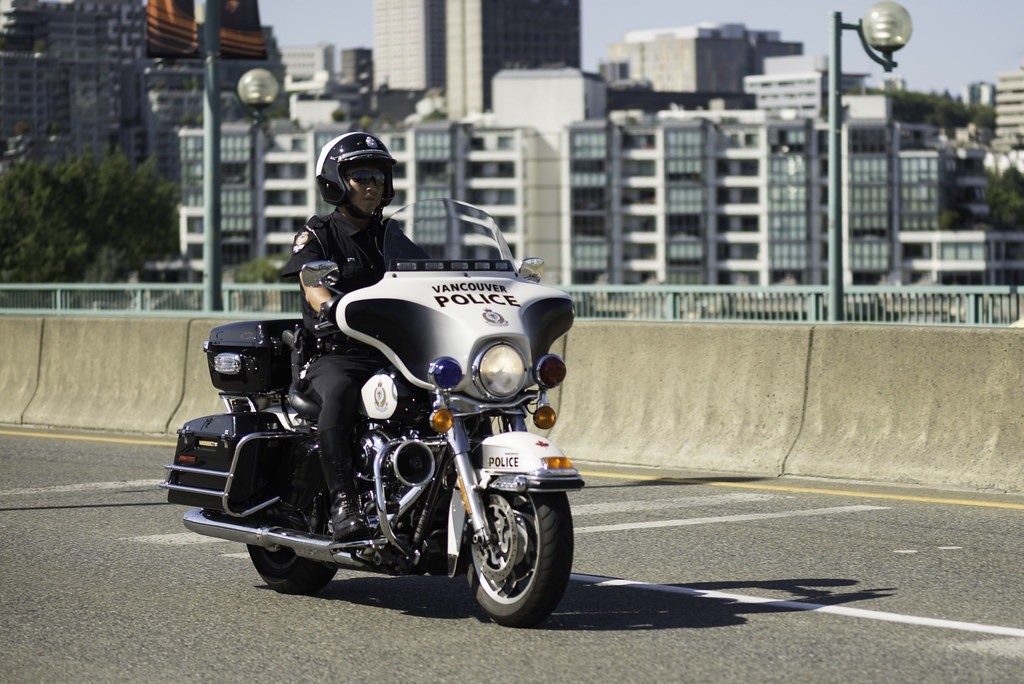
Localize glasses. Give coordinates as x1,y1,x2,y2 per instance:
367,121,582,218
346,170,386,187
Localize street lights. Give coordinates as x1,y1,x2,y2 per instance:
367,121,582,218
237,65,277,272
825,0,913,326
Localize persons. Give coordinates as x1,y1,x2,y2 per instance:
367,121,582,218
286,131,426,542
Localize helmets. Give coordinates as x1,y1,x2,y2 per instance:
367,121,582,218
316,133,396,206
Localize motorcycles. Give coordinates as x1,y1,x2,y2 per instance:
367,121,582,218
160,196,585,631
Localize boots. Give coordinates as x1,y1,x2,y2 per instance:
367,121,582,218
318,428,370,538
